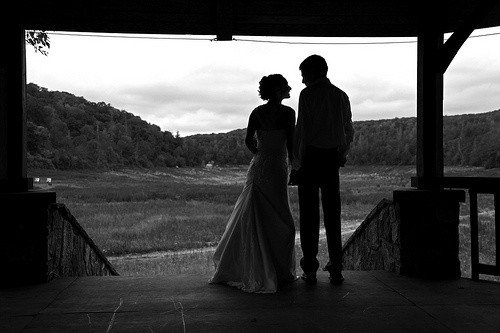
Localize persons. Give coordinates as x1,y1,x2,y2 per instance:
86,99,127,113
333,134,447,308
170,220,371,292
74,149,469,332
288,55,355,284
208,74,298,295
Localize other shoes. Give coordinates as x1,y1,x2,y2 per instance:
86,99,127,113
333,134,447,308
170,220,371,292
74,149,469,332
301,272,317,286
329,276,345,286
